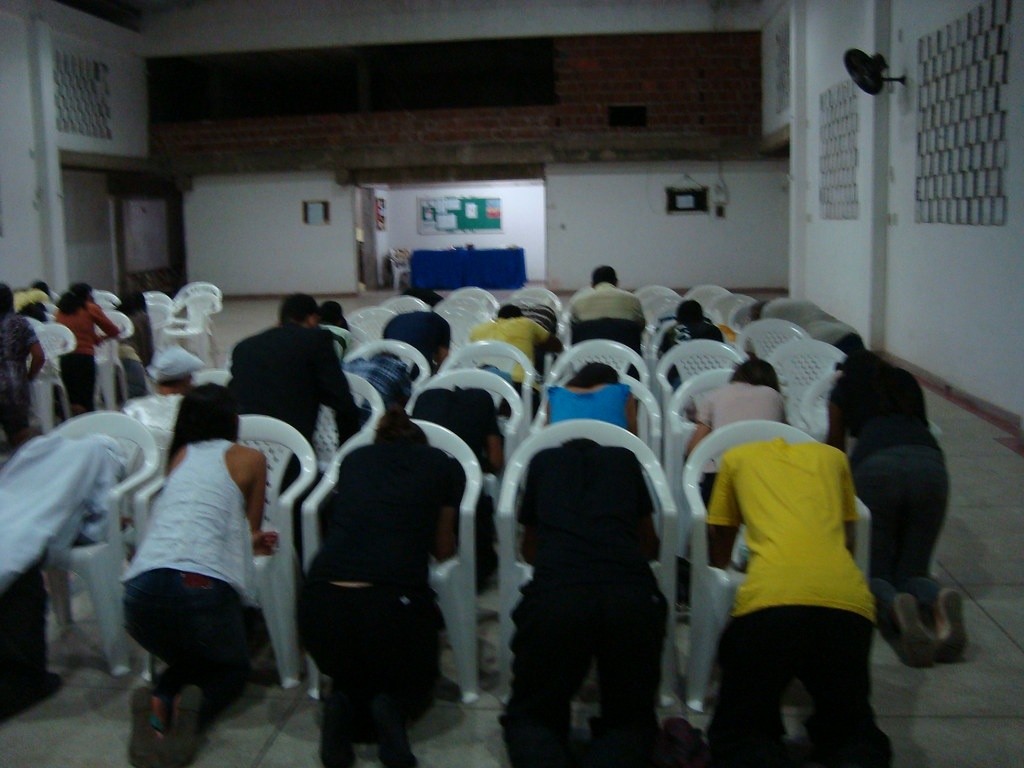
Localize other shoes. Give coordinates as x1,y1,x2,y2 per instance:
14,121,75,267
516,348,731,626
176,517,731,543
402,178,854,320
893,590,936,667
125,685,204,768
932,588,969,662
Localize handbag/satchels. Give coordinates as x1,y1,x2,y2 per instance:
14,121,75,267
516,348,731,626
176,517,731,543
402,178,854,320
654,713,713,768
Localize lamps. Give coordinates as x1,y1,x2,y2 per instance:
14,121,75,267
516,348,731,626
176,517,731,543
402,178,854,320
605,102,653,130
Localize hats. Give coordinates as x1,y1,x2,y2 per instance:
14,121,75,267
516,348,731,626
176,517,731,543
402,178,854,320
145,342,207,382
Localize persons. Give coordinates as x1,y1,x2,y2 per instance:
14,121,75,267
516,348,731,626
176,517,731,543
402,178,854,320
0,266,966,768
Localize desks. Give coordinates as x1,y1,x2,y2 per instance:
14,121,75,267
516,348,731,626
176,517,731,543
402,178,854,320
408,248,528,291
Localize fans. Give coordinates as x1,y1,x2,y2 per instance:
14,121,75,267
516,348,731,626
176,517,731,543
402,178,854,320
843,48,907,96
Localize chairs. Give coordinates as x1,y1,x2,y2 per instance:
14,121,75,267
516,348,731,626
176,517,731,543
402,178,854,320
18,279,943,712
390,248,411,291
